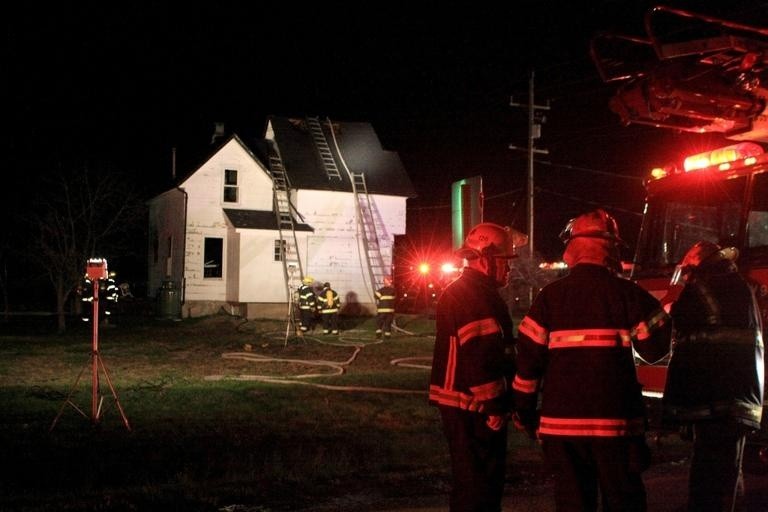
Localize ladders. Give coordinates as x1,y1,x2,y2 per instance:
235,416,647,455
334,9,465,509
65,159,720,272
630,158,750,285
351,171,389,308
306,117,343,181
269,154,305,321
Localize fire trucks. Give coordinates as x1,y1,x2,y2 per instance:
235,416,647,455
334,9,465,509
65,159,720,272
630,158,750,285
582,3,768,476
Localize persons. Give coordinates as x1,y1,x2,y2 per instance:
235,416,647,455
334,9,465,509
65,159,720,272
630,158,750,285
373,275,397,336
419,216,514,510
317,281,341,336
511,202,673,511
75,269,136,328
297,277,317,335
662,234,766,510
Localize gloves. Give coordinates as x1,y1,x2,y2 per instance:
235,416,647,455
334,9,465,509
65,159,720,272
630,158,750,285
486,414,505,432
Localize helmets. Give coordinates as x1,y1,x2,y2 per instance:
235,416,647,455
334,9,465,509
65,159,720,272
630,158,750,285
680,240,725,277
454,222,529,261
303,276,314,285
383,277,393,287
559,208,623,265
